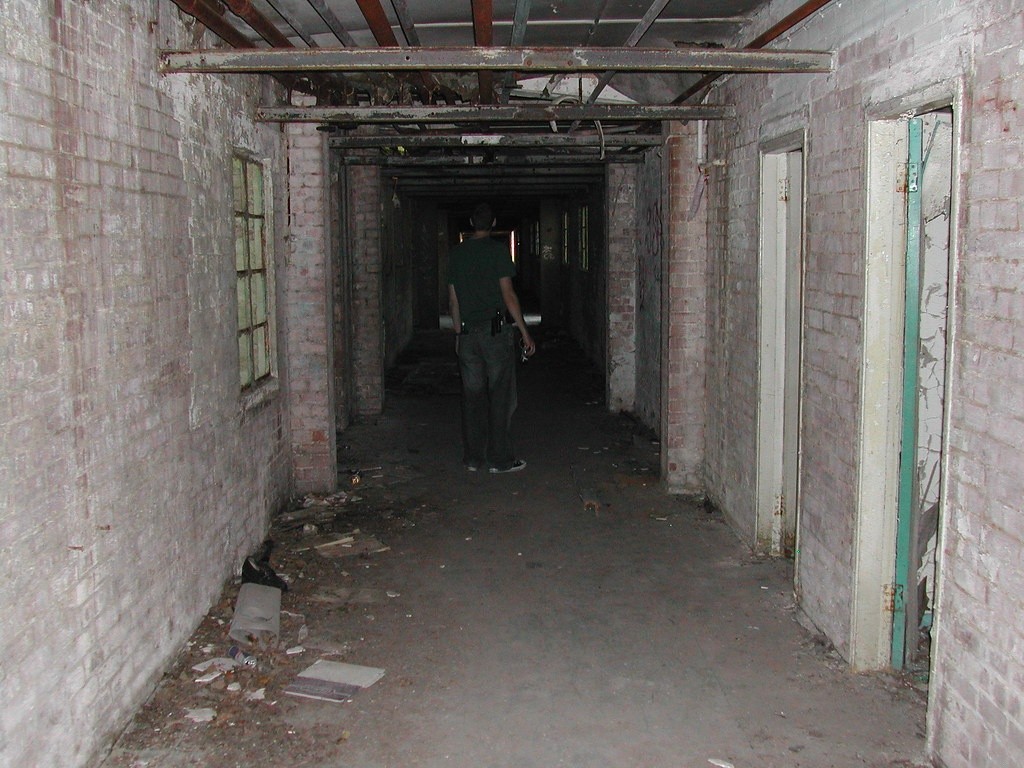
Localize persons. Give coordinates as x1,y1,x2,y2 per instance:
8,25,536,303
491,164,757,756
448,202,536,472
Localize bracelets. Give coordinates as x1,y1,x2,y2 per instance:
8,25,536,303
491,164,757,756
455,333,460,336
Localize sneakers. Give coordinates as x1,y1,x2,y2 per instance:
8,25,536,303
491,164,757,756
464,455,482,471
489,459,526,473
